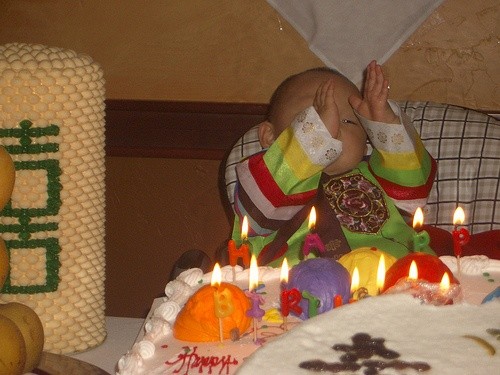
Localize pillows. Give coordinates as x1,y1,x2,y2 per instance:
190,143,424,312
225,99,499,233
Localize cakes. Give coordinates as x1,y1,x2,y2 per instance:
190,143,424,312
114,251,500,375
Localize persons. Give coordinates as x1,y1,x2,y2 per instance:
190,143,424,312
231,59,440,269
